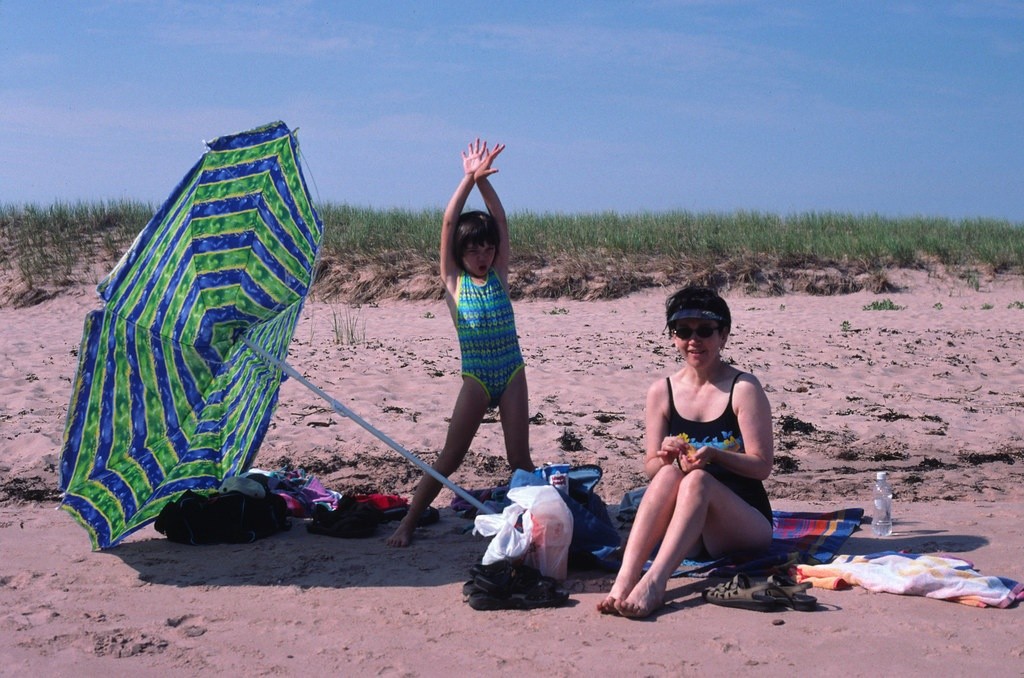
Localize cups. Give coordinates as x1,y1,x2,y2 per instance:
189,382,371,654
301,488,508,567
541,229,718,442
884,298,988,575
544,464,570,496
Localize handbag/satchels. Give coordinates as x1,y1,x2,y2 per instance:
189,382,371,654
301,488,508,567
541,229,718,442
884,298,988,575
471,463,621,582
153,473,293,545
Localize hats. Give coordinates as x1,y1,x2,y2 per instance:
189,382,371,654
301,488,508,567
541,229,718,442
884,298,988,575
667,308,727,325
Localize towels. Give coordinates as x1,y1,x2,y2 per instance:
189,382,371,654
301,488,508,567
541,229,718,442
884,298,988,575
786,543,1024,609
585,499,864,579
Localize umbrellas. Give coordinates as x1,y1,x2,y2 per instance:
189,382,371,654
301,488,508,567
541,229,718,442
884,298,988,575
54,118,499,553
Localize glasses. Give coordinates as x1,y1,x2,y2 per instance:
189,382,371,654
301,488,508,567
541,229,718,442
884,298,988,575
671,323,721,340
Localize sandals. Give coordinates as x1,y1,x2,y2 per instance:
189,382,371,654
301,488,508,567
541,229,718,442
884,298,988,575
702,572,817,613
462,561,570,611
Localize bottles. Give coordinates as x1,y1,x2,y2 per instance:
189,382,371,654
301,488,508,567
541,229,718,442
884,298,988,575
872,472,893,536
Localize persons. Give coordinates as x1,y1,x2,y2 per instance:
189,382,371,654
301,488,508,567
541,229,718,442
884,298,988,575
597,285,775,618
387,138,537,548
